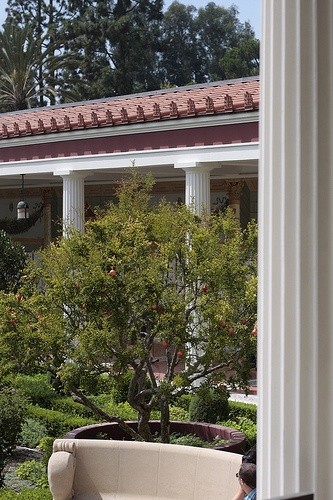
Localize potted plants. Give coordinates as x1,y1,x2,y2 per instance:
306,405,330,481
0,161,258,451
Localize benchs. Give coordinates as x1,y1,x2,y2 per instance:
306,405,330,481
46,438,247,500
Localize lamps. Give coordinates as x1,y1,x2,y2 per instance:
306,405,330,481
16,173,30,221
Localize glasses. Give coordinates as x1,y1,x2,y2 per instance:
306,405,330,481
235,472,241,478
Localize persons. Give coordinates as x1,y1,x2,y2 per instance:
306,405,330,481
233,462,257,500
